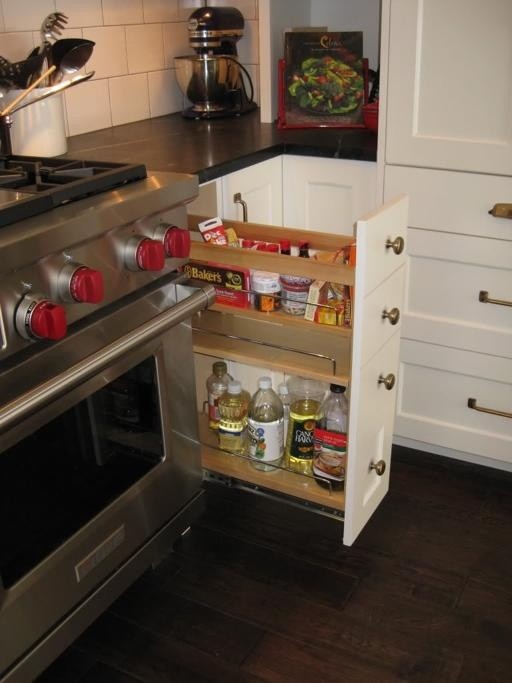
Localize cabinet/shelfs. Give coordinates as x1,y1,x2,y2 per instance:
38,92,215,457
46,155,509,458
186,155,415,544
381,163,512,474
375,1,512,176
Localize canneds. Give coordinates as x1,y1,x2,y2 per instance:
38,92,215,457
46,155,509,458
278,275,313,315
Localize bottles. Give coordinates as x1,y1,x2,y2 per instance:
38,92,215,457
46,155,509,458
207,360,233,433
285,377,325,478
216,381,252,457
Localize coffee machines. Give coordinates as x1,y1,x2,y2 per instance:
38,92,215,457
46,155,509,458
171,3,260,125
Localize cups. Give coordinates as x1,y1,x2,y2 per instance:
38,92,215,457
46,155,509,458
2,77,70,159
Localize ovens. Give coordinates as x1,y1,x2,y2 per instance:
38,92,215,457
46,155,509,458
0,206,223,683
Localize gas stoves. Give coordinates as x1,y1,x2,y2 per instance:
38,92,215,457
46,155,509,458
0,151,161,236
